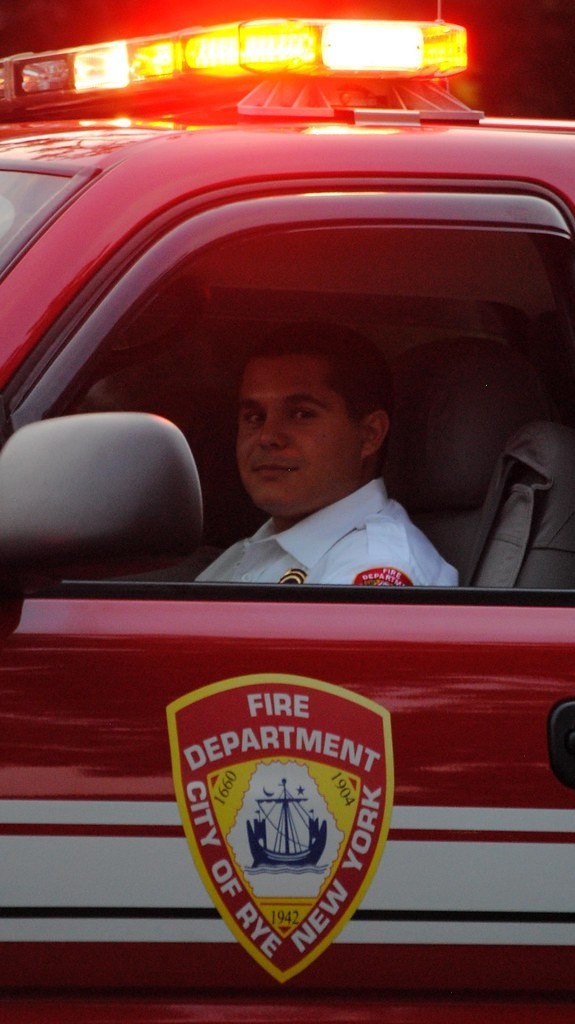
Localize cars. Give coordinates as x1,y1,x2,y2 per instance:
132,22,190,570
0,18,575,1024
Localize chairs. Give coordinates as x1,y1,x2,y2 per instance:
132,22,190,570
375,330,575,590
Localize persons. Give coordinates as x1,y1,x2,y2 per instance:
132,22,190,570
188,315,459,590
